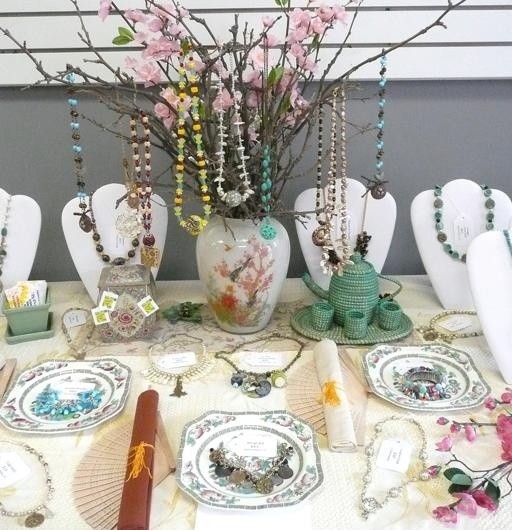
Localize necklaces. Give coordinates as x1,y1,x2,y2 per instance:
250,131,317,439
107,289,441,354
416,307,485,344
208,432,295,494
0,195,13,294
142,332,215,399
60,306,97,360
30,374,106,419
434,182,495,263
503,229,512,257
1,440,55,528
358,415,428,520
214,331,305,398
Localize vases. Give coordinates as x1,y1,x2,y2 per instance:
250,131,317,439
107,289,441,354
195,211,291,334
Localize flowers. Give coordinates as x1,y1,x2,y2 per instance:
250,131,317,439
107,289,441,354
430,386,512,529
0,0,471,239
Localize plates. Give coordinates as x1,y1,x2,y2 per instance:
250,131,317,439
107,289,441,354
360,341,492,411
0,358,132,434
174,409,324,511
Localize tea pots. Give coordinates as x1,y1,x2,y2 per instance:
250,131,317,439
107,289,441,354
302,254,403,326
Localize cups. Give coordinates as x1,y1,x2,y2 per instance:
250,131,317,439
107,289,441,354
344,309,367,339
2,286,52,335
379,301,401,331
311,303,334,331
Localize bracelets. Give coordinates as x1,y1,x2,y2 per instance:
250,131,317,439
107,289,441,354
400,366,445,400
